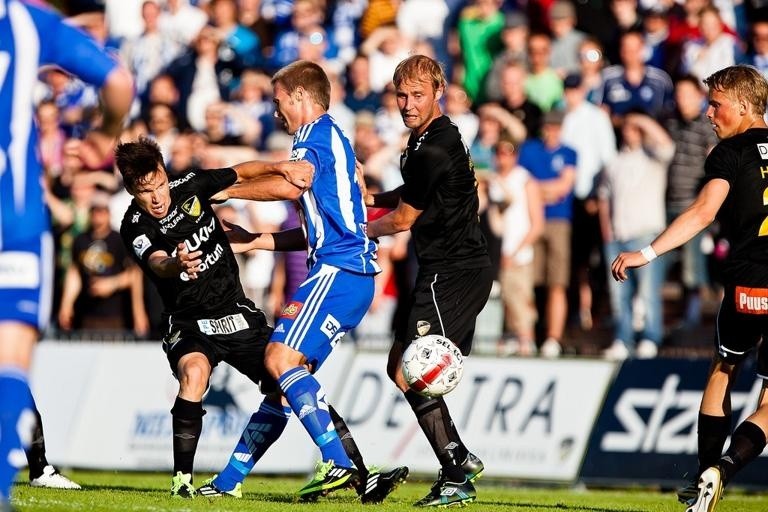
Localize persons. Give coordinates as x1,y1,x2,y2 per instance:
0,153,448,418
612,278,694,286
0,0,768,510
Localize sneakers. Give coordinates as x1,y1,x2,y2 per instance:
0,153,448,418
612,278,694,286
194,474,242,499
358,465,409,504
170,471,200,501
413,468,477,508
459,452,484,482
297,458,361,501
30,465,81,490
678,467,724,512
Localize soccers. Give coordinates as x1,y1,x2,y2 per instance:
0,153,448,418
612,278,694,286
402,334,464,397
